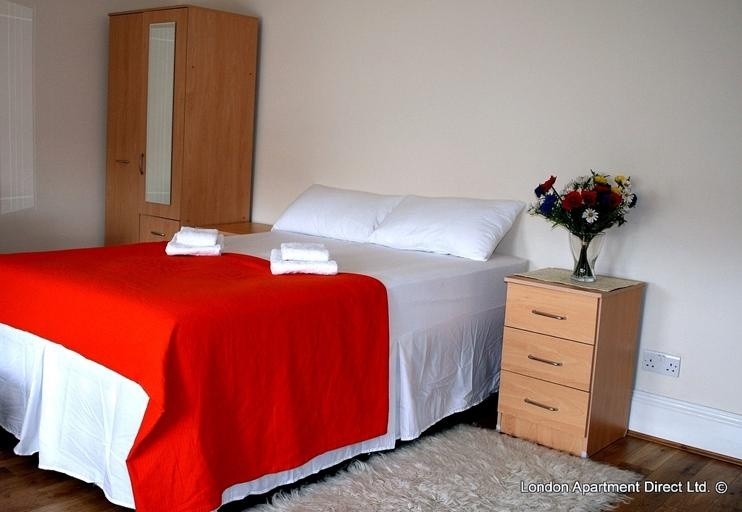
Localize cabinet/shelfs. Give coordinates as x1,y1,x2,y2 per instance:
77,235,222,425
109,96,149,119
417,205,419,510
104,7,258,248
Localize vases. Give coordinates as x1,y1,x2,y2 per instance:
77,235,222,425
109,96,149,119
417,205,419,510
566,229,607,284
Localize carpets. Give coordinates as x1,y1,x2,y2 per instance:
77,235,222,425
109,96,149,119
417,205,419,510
242,422,645,512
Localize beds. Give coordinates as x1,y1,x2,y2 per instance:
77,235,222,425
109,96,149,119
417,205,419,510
1,226,529,510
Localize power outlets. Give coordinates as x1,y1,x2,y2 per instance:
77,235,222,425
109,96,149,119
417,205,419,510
642,348,681,380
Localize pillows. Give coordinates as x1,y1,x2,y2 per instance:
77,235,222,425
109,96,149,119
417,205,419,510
268,183,528,263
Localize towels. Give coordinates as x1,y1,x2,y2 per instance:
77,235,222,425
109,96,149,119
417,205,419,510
164,223,340,278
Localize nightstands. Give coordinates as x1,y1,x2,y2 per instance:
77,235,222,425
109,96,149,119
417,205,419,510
194,220,274,242
495,268,649,459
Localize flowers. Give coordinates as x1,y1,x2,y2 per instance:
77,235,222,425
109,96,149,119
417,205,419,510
526,166,641,276
